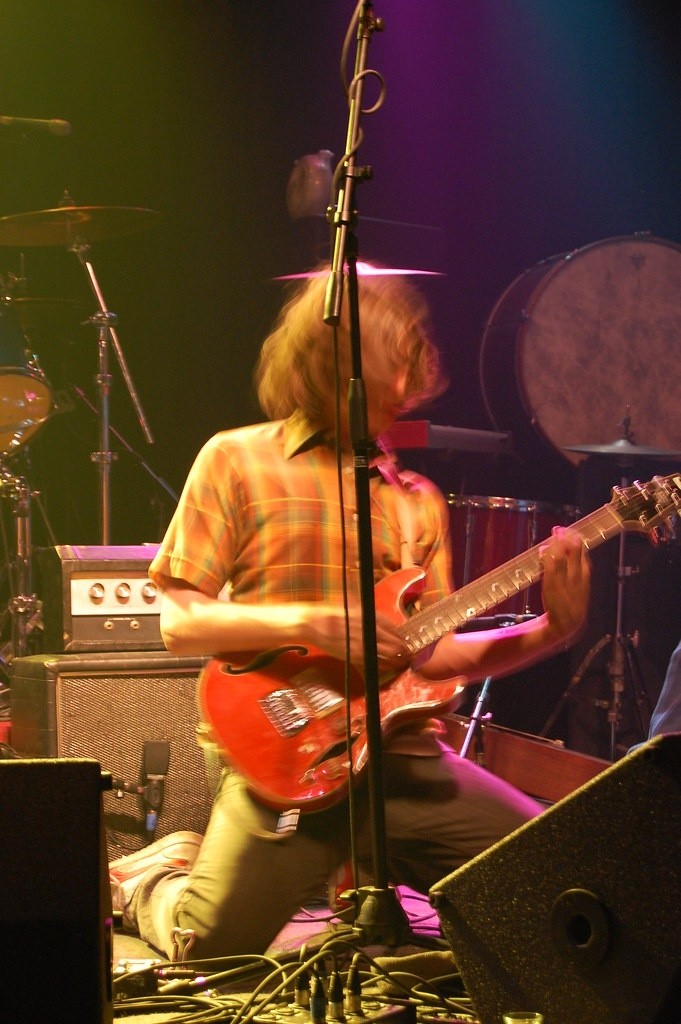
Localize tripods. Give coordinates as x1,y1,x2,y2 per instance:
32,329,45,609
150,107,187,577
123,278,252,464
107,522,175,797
152,0,450,999
541,465,650,762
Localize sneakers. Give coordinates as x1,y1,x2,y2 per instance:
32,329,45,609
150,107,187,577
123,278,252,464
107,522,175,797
108,830,203,915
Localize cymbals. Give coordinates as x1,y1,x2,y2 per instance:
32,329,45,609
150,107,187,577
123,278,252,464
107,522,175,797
271,260,444,280
0,290,91,323
0,205,165,249
559,442,681,460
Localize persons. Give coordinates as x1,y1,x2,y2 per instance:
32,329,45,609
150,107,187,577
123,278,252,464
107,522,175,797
105,258,592,972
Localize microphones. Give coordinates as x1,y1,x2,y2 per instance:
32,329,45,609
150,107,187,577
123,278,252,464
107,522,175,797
142,739,169,844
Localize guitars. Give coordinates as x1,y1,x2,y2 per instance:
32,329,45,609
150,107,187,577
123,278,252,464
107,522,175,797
193,469,681,819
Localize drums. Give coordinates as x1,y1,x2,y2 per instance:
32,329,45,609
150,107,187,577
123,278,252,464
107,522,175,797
477,229,681,499
0,302,54,462
442,491,601,631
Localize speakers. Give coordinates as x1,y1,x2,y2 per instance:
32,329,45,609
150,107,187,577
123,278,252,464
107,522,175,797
12,653,225,864
429,732,681,1024
0,757,117,1024
